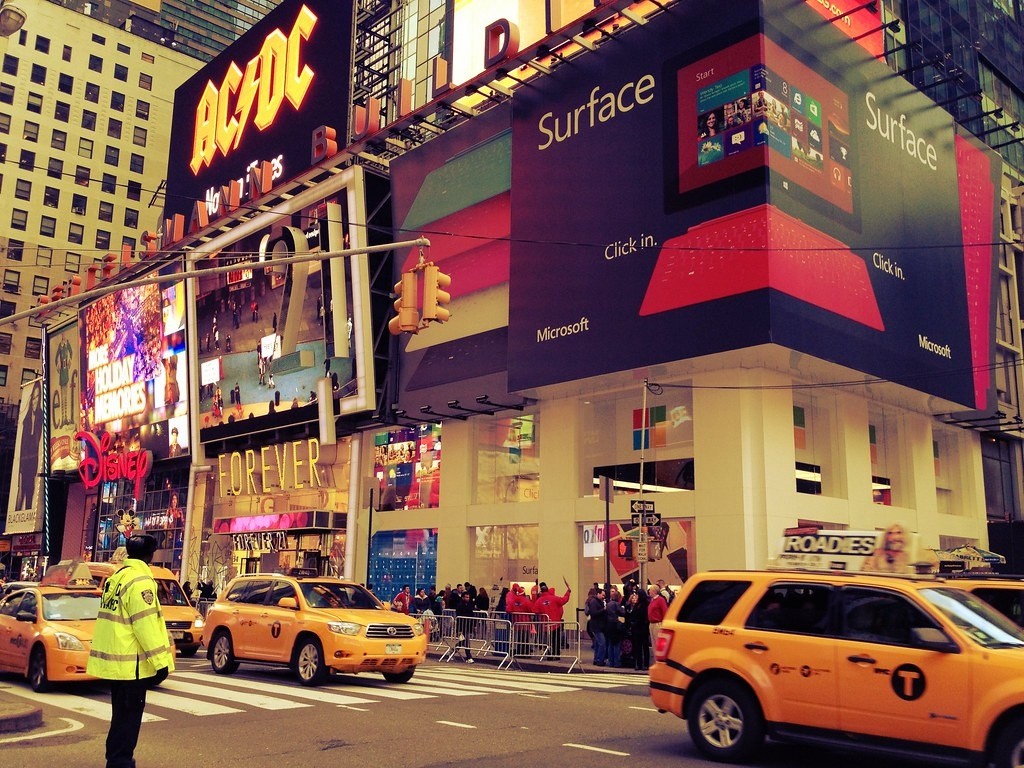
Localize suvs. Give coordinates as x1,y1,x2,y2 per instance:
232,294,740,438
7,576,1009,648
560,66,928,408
649,568,1024,768
202,568,428,687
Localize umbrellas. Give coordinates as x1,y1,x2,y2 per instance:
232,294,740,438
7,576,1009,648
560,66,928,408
947,542,1006,569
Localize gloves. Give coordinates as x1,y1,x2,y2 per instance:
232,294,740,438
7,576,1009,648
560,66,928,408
154,668,167,683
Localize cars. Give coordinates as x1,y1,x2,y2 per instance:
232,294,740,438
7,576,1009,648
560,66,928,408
0,561,103,693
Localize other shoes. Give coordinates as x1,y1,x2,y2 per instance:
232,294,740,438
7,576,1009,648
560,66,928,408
465,659,474,664
448,651,453,661
593,660,606,666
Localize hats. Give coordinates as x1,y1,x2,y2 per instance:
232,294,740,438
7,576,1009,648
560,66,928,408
435,596,443,602
516,587,525,595
125,534,158,556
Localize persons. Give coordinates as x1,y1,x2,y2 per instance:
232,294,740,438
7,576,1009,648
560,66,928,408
585,578,675,672
317,292,324,324
13,380,43,511
231,382,240,404
87,534,175,768
197,579,217,600
181,581,192,601
724,91,791,133
861,523,911,573
495,579,571,661
211,387,224,418
392,581,490,663
351,583,380,608
163,493,185,539
55,333,73,428
324,358,338,390
198,300,278,387
169,427,181,457
697,111,723,142
310,391,316,400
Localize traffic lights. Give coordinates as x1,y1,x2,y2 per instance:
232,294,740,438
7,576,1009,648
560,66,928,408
648,540,661,560
618,540,632,558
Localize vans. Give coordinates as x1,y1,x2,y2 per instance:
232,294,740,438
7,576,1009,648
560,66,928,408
42,547,205,656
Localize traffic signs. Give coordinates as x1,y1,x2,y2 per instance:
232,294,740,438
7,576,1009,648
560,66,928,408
630,499,661,527
